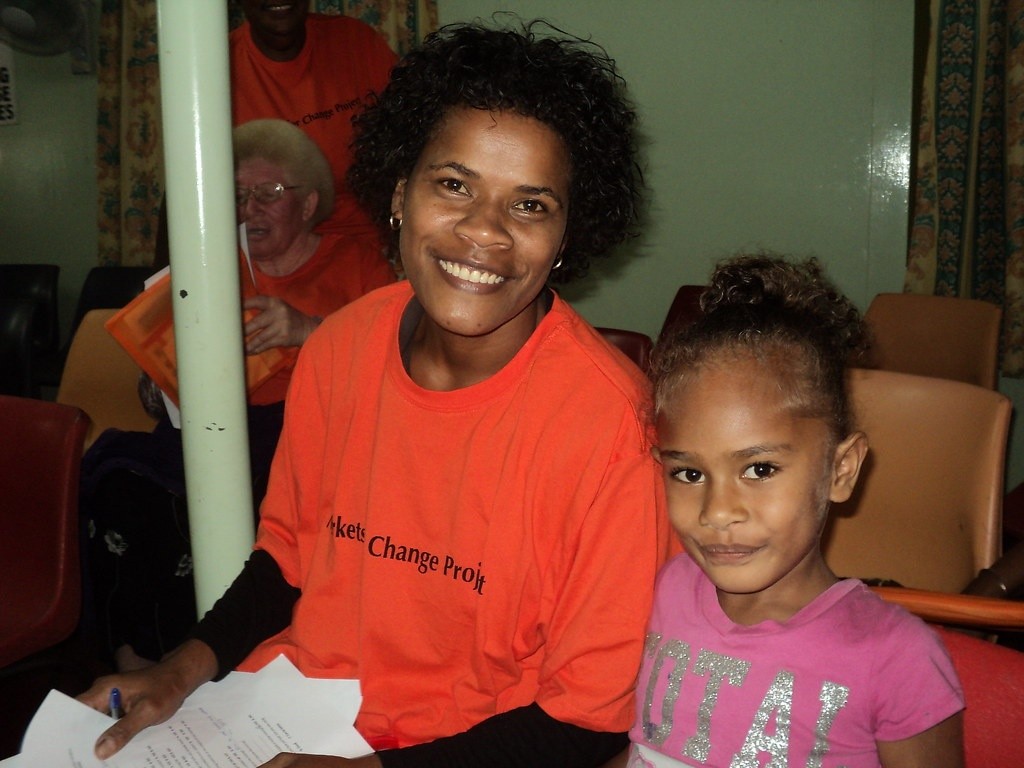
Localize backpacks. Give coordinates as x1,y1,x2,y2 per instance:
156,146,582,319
77,433,266,664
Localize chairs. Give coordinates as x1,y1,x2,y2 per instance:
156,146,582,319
53,308,159,458
820,367,1014,594
67,265,165,349
0,394,90,737
0,264,61,399
863,291,1001,390
868,584,1024,768
656,283,711,359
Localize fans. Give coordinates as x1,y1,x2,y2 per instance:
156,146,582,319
0,0,97,75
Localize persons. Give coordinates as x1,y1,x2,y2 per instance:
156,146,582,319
78,118,399,673
67,10,685,768
139,1,406,294
603,254,969,768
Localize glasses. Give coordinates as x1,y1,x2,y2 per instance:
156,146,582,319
235,181,305,205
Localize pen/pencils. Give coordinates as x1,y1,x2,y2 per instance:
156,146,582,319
108,687,121,719
